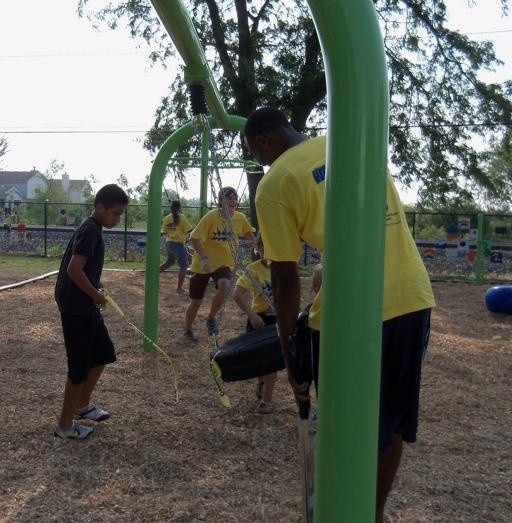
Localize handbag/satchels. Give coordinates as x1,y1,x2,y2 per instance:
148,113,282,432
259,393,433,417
210,323,297,409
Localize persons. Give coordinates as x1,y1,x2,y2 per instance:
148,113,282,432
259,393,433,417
54,184,130,440
183,187,257,341
311,265,323,294
160,200,192,292
234,229,278,413
242,106,437,523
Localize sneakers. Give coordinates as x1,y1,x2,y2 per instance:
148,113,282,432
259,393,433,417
256,382,264,399
160,265,164,273
207,316,219,336
54,422,94,440
184,330,197,344
73,403,110,422
255,400,273,414
177,288,189,293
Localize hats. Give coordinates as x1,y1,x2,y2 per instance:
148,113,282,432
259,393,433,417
217,187,238,208
